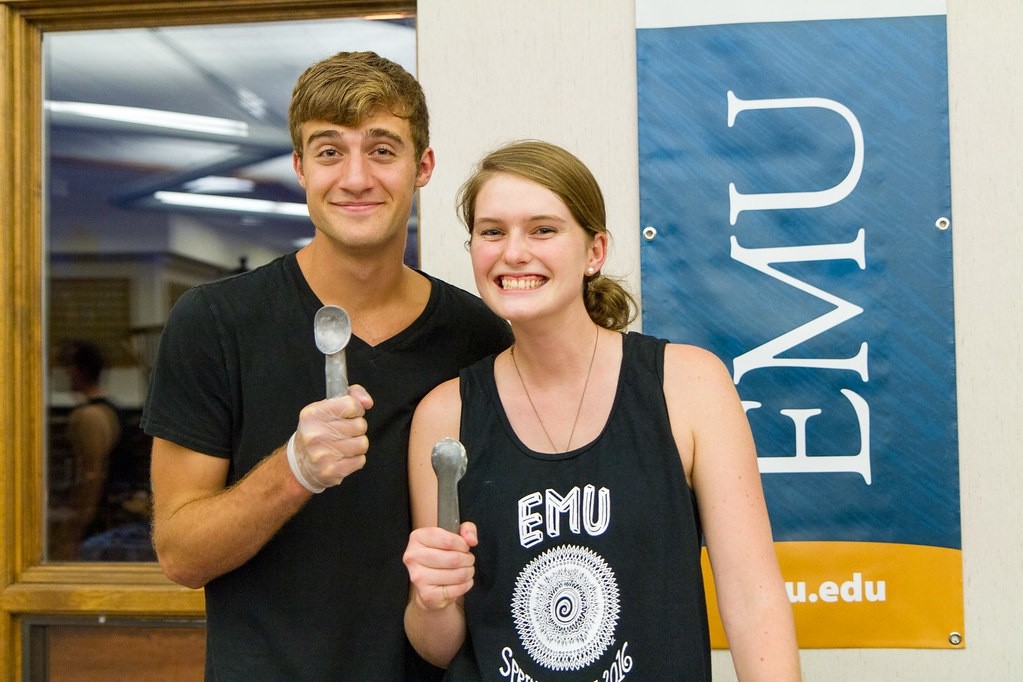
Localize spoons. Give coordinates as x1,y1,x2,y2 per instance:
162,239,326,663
431,437,469,535
313,305,352,401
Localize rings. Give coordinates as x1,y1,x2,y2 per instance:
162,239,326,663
442,585,451,602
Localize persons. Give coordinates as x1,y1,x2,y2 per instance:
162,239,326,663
137,49,514,681
50,338,125,564
404,140,804,682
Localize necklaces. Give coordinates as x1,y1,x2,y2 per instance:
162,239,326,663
509,323,600,456
